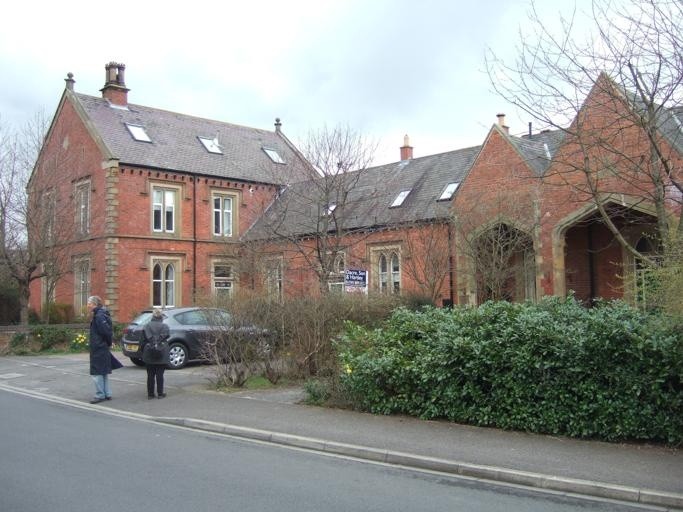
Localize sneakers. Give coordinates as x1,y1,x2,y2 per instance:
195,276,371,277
146,392,167,400
89,396,112,405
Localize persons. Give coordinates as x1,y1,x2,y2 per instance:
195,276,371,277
137,309,170,400
86,295,124,404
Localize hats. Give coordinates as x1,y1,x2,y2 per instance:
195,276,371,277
150,308,168,322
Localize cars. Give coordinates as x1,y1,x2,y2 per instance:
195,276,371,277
121,307,271,369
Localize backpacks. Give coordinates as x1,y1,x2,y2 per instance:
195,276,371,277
146,335,165,362
